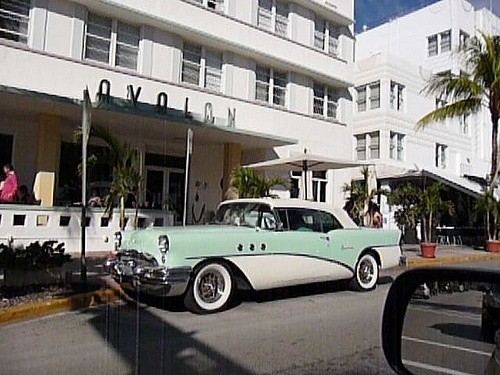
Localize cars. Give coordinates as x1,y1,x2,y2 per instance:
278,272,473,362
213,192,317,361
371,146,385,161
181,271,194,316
111,198,402,316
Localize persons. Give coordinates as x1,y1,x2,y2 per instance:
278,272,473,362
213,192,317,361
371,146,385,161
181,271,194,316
289,214,311,231
88,187,102,207
18,185,32,203
0,162,18,201
367,202,383,228
343,200,360,226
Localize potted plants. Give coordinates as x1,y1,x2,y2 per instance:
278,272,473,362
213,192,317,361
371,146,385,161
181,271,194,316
389,178,455,258
474,186,500,254
73,119,144,258
